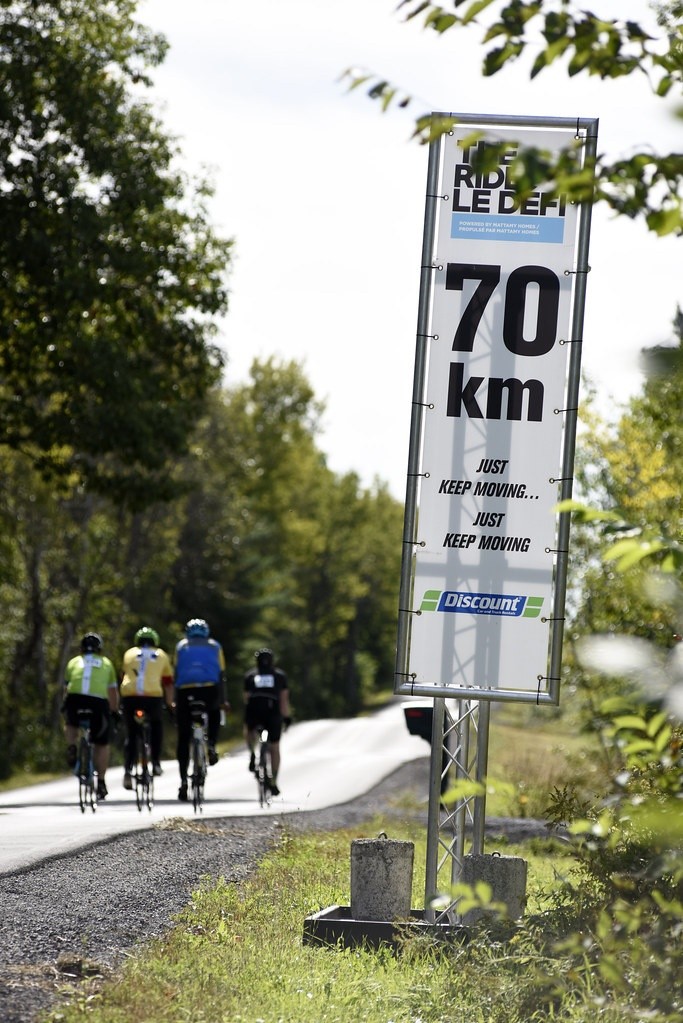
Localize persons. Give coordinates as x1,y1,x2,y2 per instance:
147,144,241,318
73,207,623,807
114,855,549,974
120,626,176,791
60,632,120,797
173,618,230,803
242,648,292,796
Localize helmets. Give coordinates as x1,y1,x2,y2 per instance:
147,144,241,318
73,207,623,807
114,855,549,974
133,626,160,647
78,633,103,648
256,649,275,663
184,618,209,636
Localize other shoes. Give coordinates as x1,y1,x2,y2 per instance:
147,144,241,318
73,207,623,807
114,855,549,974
121,772,132,791
248,753,257,774
208,751,219,766
66,759,79,776
152,765,163,776
269,781,280,796
96,782,109,796
177,783,189,802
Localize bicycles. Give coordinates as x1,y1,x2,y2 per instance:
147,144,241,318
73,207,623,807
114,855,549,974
117,702,171,811
176,698,230,813
70,706,121,813
248,720,291,810
